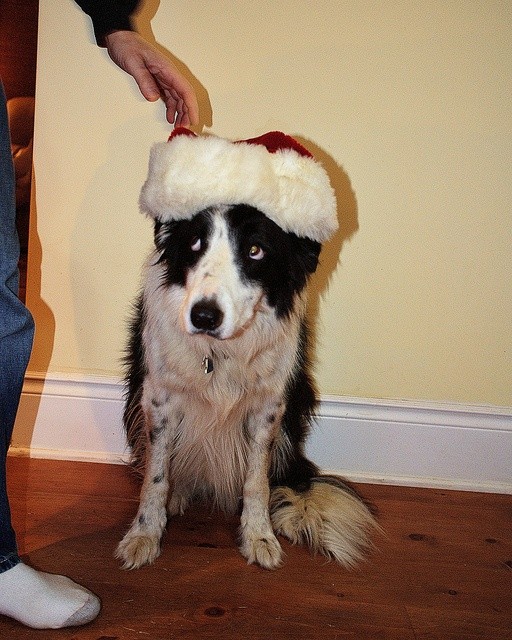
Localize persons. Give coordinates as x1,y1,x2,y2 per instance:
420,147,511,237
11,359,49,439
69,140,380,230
1,0,202,631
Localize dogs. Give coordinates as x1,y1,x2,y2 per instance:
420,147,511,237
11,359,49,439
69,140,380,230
111,128,387,571
